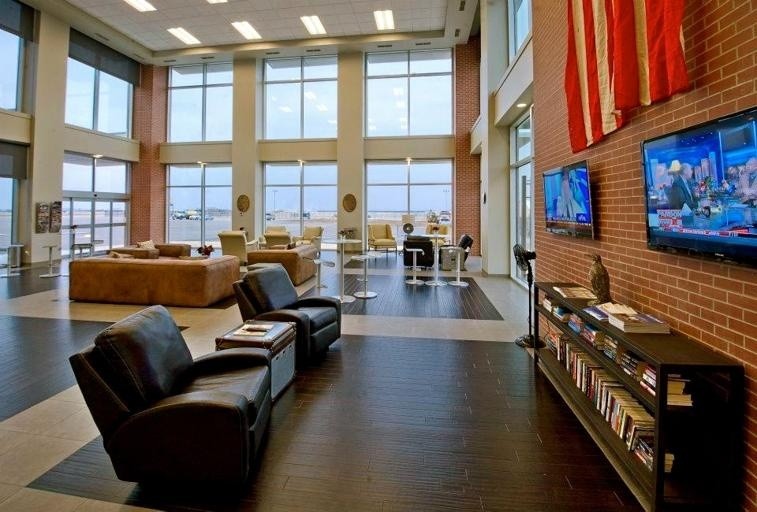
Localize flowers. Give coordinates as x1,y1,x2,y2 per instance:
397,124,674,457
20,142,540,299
339,226,357,239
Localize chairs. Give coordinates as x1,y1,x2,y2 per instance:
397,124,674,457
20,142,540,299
68,303,272,511
218,225,323,261
233,263,340,368
368,223,474,267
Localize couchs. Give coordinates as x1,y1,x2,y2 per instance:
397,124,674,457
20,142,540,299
69,241,324,307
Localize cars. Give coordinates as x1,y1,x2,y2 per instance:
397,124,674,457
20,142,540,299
649,189,660,204
696,201,722,217
266,213,276,221
303,210,310,219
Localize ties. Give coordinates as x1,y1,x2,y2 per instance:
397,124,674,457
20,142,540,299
566,204,570,219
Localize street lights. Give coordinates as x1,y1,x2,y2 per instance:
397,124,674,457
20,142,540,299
442,188,450,210
269,190,278,211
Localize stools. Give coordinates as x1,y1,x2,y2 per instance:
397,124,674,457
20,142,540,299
0,244,60,278
351,253,381,299
313,259,335,298
448,248,470,286
405,242,424,287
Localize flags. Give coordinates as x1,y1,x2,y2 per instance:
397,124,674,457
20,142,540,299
564,0,694,155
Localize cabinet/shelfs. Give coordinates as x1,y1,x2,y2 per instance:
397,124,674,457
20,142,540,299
534,283,745,512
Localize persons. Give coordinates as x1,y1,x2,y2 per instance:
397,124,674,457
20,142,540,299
667,162,695,209
568,167,586,212
722,165,739,195
736,158,756,206
555,176,583,217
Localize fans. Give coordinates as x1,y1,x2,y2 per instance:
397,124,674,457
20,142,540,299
513,243,546,348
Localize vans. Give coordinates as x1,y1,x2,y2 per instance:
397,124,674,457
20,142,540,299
439,215,451,226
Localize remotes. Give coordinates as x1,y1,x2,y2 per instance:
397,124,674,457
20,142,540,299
247,327,267,332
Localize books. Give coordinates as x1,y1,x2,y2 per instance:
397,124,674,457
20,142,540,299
232,324,275,336
537,286,693,473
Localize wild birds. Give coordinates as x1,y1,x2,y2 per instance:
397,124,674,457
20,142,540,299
584,252,617,306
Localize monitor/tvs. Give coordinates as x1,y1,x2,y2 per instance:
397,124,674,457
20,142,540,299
542,159,595,240
642,105,757,269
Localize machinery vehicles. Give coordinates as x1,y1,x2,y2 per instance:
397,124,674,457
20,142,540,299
172,209,213,221
